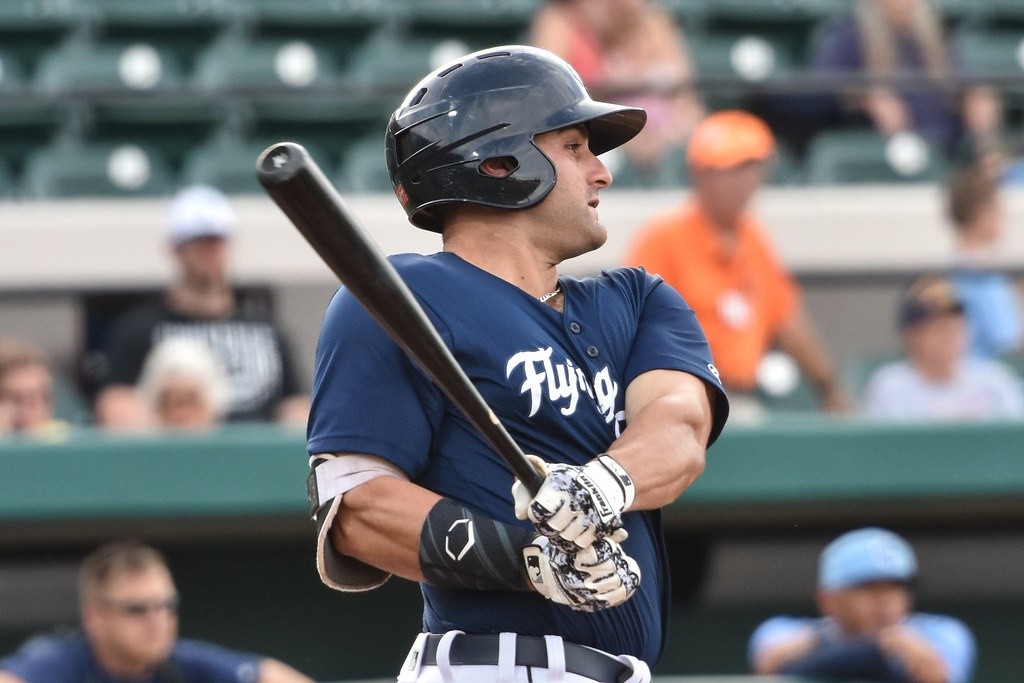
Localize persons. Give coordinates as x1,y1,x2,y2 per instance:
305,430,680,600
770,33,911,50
0,186,317,683
306,45,730,683
747,527,976,683
529,0,1024,411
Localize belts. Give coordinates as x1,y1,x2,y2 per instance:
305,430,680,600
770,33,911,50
419,630,634,683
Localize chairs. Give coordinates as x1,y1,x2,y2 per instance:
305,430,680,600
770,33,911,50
0,0,1024,203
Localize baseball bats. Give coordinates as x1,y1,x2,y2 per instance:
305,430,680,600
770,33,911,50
251,141,545,506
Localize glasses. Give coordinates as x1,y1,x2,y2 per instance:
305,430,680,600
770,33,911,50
93,597,178,616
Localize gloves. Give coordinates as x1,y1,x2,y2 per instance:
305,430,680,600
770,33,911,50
522,528,642,612
512,454,635,549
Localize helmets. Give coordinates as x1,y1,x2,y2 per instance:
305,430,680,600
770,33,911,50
383,46,646,230
819,527,916,589
162,185,232,245
685,111,776,170
899,278,967,324
